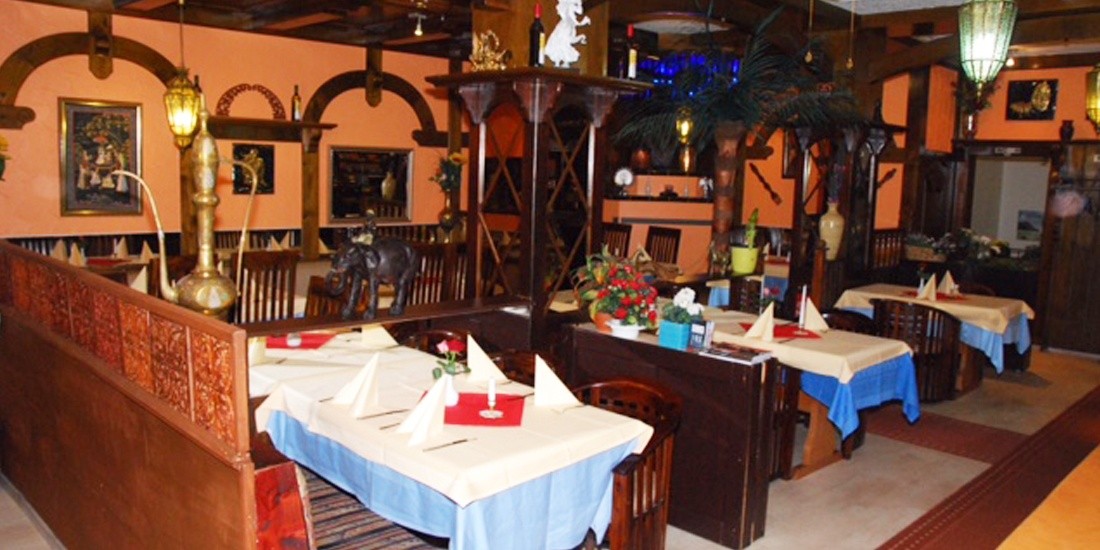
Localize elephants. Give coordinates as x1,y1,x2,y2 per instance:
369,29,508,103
325,235,423,321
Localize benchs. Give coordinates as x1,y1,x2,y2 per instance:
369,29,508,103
251,433,436,550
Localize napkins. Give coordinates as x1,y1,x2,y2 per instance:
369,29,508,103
534,352,583,410
918,270,960,301
467,334,511,386
743,301,775,343
336,351,416,419
804,298,829,332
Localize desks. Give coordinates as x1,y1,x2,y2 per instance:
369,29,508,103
655,297,920,480
252,342,655,550
835,283,1039,395
84,254,147,291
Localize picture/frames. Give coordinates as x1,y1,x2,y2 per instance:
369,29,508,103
58,96,143,217
327,144,415,223
782,127,797,179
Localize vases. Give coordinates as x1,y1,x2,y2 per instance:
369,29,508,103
659,320,702,349
438,187,458,244
818,202,846,260
706,118,748,277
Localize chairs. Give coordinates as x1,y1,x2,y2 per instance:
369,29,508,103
794,298,960,403
402,327,682,549
602,222,681,264
148,243,467,323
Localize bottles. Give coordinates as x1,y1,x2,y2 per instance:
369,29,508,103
529,4,546,67
193,75,202,94
291,84,301,121
623,22,638,80
381,172,396,200
1059,120,1074,140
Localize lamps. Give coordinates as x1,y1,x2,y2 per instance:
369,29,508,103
1084,61,1100,135
958,0,1019,104
163,0,202,154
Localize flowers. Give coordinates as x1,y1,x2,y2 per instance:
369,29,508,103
929,226,992,280
430,150,464,190
432,335,472,379
567,244,706,331
826,162,846,203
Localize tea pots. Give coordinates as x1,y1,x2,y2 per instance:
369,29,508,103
111,94,258,322
